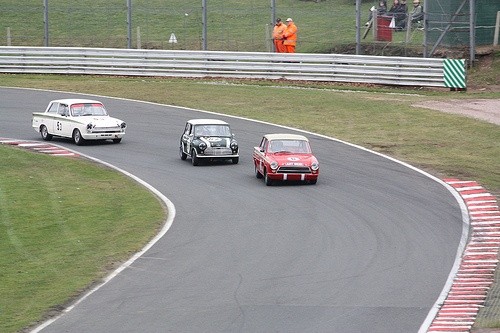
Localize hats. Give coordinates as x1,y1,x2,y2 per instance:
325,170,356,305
286,17,292,21
276,18,281,22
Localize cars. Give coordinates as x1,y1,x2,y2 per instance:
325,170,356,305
252,133,319,186
31,98,128,145
178,118,240,166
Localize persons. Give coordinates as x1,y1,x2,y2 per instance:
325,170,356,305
365,0,424,28
273,18,297,53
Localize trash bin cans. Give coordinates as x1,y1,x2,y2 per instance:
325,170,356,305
377,15,393,41
